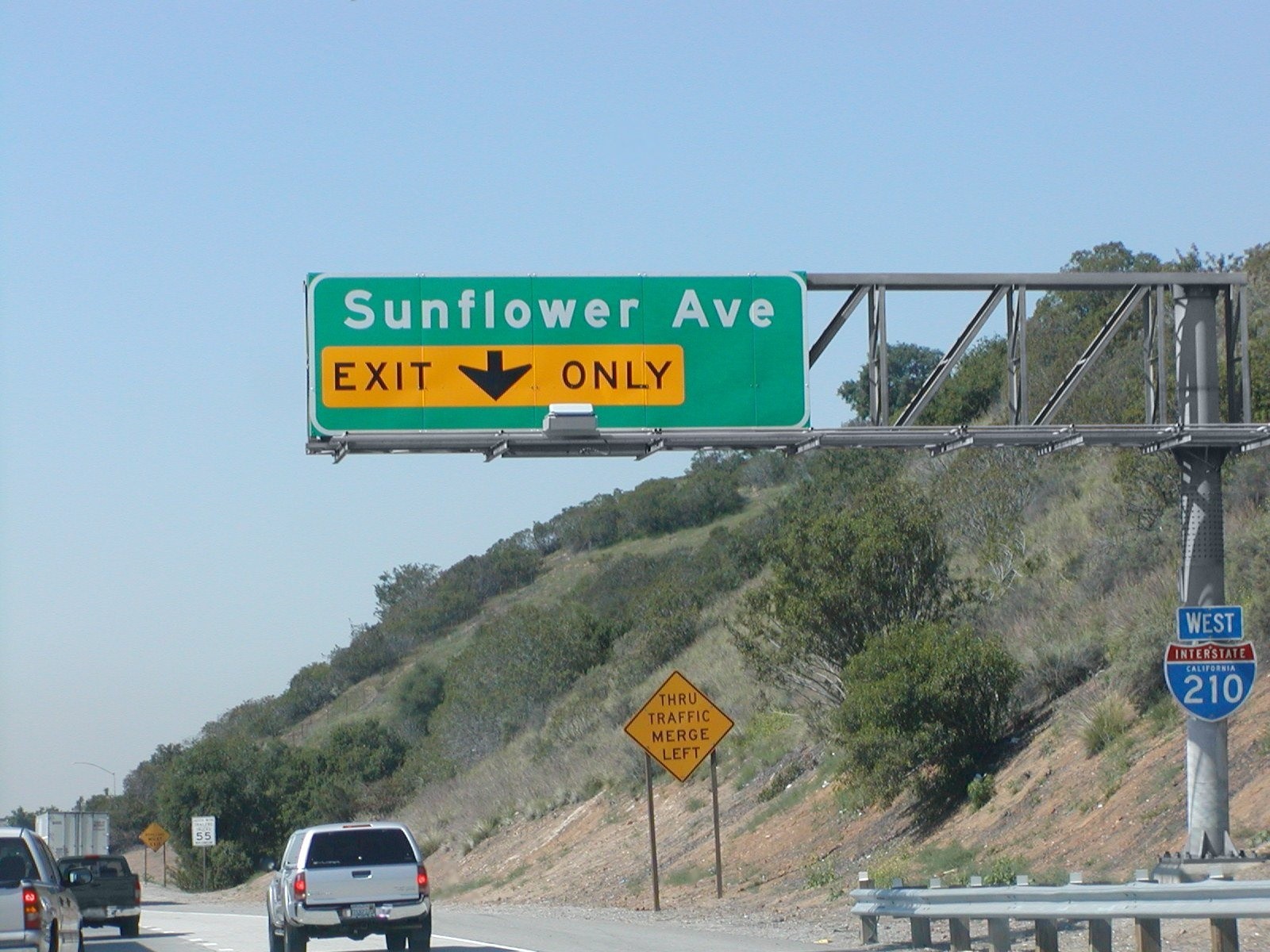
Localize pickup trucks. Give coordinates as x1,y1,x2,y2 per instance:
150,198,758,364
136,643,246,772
56,854,141,939
0,826,94,952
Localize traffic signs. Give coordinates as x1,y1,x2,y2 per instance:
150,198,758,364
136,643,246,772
301,269,814,453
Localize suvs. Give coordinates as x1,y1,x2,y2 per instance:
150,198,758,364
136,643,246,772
265,822,432,952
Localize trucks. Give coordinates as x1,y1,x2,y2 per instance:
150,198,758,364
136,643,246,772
35,810,111,860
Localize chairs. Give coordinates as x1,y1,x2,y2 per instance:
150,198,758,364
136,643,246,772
0,856,26,881
100,868,117,877
